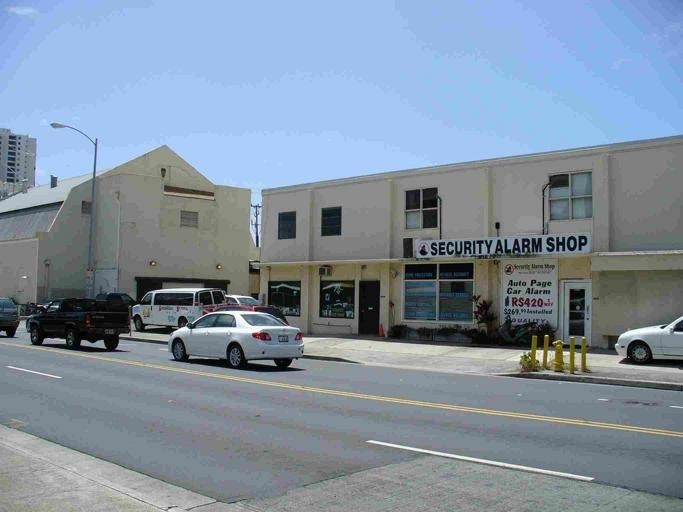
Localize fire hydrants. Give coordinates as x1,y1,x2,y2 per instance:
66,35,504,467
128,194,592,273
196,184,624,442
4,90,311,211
553,340,565,374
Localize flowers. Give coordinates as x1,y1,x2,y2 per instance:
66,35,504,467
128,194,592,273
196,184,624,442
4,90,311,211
468,295,495,322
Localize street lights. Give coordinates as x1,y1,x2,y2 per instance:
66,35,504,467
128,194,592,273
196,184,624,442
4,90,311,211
50,123,97,271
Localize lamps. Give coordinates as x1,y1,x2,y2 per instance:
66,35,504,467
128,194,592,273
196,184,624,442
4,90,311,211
149,260,157,266
215,264,222,270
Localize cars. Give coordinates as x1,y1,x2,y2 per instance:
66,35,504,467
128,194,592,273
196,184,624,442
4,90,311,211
614,316,683,365
224,294,261,305
0,297,20,337
212,303,289,327
167,311,304,369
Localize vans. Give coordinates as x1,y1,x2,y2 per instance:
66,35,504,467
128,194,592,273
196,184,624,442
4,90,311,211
132,287,225,332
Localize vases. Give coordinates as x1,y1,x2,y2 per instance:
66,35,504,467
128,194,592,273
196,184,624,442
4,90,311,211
478,322,489,335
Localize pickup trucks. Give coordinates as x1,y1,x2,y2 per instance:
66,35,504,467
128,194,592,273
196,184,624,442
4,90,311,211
25,293,137,350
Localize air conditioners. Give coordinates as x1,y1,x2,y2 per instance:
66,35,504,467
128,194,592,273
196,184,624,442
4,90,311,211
318,267,333,277
